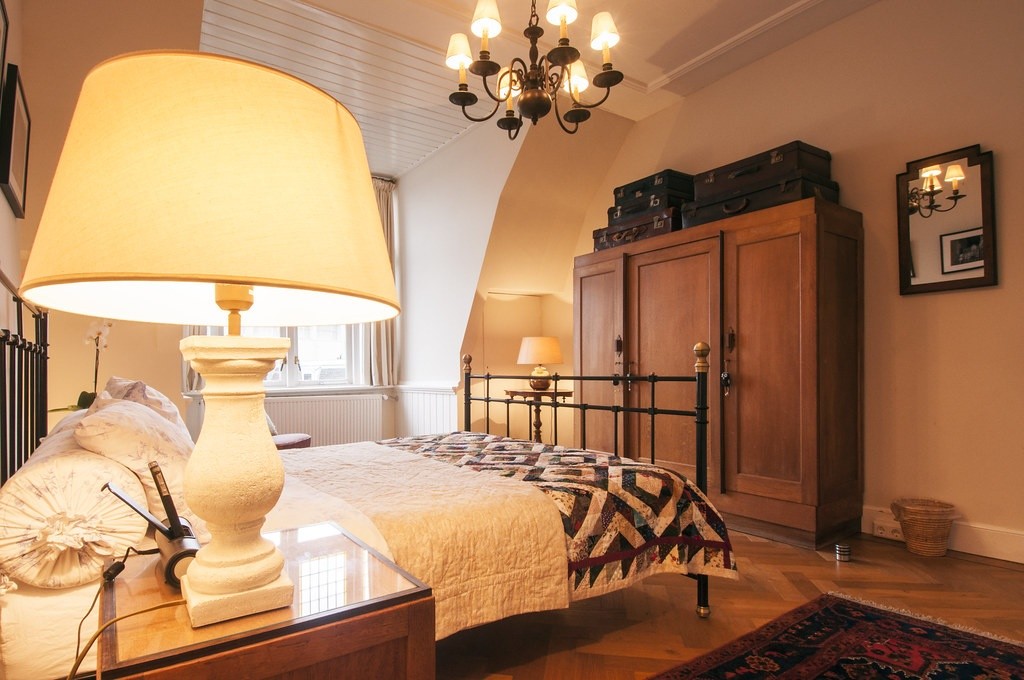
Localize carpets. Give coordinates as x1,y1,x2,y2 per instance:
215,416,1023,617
645,588,1024,680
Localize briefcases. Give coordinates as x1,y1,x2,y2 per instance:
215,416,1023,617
593,140,839,253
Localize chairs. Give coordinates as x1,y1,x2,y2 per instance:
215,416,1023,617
264,412,312,450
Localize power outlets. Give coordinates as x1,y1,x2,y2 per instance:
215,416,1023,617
873,520,906,542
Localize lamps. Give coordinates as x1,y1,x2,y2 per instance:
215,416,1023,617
516,335,562,390
445,0,625,141
908,164,967,219
17,49,401,629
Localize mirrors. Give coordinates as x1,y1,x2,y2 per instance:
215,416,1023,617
895,144,999,296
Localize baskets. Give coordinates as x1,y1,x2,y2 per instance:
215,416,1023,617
891,497,961,556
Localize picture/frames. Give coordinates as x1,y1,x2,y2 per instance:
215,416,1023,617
0,63,31,220
939,226,984,275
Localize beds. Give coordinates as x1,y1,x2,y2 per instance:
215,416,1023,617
0,271,740,680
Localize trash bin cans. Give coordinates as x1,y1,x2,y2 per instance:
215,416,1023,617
890,498,955,557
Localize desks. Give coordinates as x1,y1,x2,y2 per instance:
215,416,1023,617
504,389,574,443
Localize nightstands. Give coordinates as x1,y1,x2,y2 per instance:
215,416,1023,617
96,518,437,680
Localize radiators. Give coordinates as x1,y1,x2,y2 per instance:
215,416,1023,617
265,394,383,447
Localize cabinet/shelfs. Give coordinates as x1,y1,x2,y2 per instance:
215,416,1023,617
573,196,865,551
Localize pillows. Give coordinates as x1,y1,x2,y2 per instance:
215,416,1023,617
73,388,207,535
1,409,150,589
103,376,193,441
